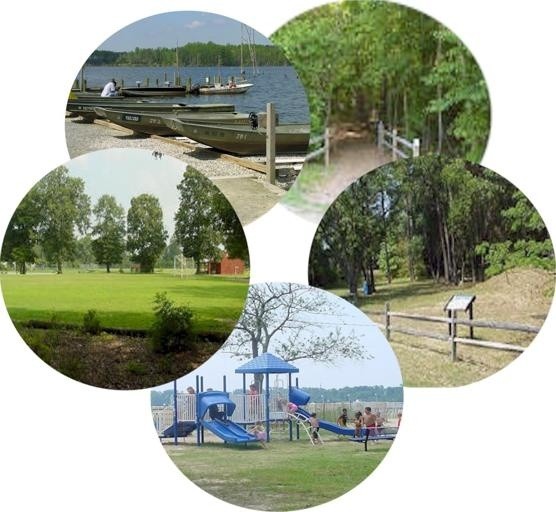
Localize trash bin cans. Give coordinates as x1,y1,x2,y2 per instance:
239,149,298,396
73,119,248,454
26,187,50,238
364,280,372,295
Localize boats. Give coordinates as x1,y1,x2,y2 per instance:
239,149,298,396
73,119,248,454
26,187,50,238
66,67,311,156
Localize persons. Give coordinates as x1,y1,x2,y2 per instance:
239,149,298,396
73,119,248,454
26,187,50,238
281,399,297,421
244,420,267,450
335,407,401,445
184,387,195,421
99,77,120,97
301,412,320,445
245,385,262,421
207,387,224,421
272,392,285,426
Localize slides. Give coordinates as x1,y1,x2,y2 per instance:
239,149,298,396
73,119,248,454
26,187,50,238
197,418,259,443
293,406,368,435
